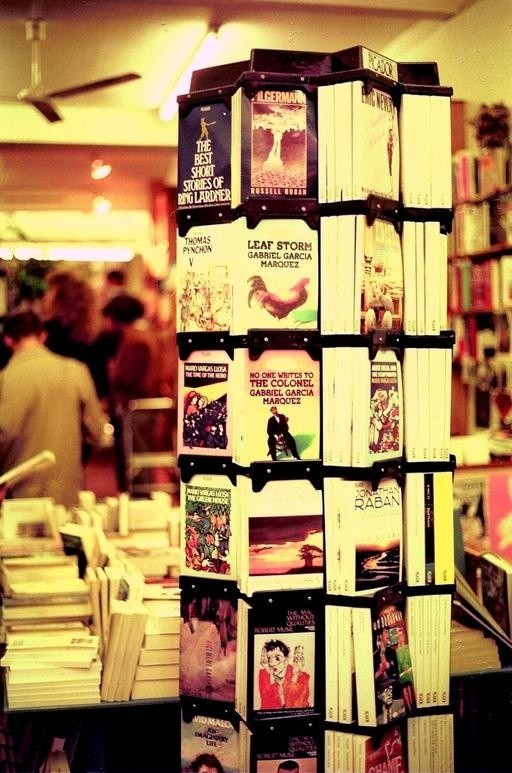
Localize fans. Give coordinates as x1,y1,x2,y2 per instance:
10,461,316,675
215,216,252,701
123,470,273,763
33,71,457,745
1,11,142,124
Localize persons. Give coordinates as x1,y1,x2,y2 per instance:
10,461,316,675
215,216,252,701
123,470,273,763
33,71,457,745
266,407,300,461
197,396,208,411
187,390,200,416
364,265,393,336
41,281,122,400
258,639,310,709
0,286,45,371
102,296,162,492
102,270,144,326
0,309,114,511
186,502,230,574
373,626,402,716
273,760,299,773
387,129,394,176
188,752,225,773
375,678,404,724
199,118,216,141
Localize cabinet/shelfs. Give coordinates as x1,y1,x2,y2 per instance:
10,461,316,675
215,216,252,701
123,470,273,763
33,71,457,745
172,46,453,773
454,150,512,739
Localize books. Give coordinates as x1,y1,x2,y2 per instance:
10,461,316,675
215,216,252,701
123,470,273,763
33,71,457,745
324,474,404,597
325,729,407,773
324,346,402,467
230,86,320,208
237,595,325,723
451,470,511,674
176,583,236,707
405,471,454,589
316,79,401,202
181,703,249,773
177,349,233,458
455,149,512,465
249,721,324,773
402,220,449,338
176,224,233,334
325,604,416,728
178,96,232,209
401,93,452,210
233,218,319,337
402,346,453,464
233,349,322,465
408,593,452,708
320,215,403,335
0,490,181,709
180,473,237,581
236,476,326,597
409,714,454,773
